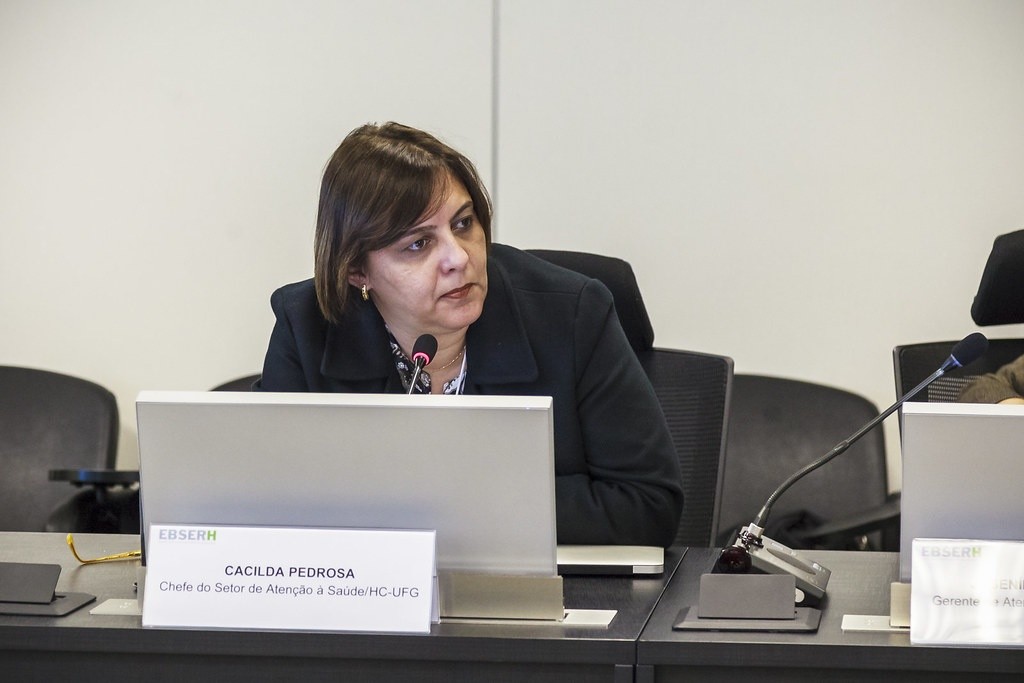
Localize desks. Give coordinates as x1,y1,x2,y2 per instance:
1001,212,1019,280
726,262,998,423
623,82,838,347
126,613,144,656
0,548,1024,683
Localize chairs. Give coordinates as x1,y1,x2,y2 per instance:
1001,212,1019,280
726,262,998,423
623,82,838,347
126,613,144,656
713,368,904,555
0,358,145,534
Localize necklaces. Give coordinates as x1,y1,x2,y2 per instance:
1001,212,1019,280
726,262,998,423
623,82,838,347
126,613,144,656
403,349,464,371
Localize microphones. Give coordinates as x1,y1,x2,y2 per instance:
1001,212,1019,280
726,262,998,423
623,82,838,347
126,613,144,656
709,332,990,606
406,333,439,394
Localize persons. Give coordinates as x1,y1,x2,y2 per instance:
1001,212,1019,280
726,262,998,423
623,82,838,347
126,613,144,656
250,121,687,550
956,353,1024,404
